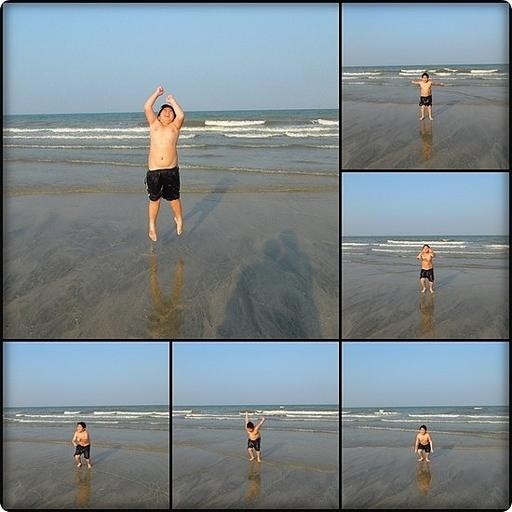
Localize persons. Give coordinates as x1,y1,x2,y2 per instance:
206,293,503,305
417,244,436,294
74,469,93,509
244,411,266,461
420,121,432,163
145,253,192,338
72,421,94,469
414,424,433,461
247,462,262,502
416,462,432,495
144,86,184,243
410,72,444,120
418,295,435,331
